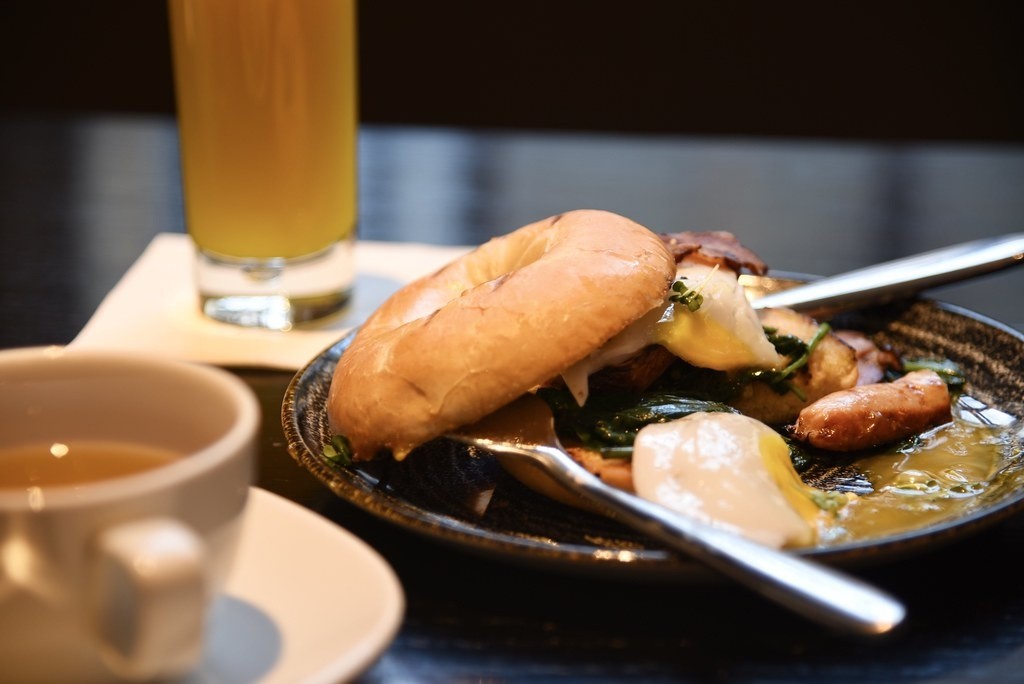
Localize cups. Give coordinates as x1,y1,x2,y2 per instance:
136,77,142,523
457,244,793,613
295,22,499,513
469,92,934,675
167,0,358,332
0,345,261,684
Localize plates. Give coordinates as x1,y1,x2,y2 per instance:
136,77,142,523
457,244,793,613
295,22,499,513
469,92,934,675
281,269,1024,583
202,486,406,684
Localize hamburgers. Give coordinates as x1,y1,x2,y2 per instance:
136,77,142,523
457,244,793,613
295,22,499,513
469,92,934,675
318,210,896,507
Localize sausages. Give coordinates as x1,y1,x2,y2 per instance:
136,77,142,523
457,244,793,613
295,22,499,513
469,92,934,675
793,370,952,446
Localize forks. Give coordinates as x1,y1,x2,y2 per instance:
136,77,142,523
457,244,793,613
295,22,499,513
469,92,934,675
437,392,908,636
